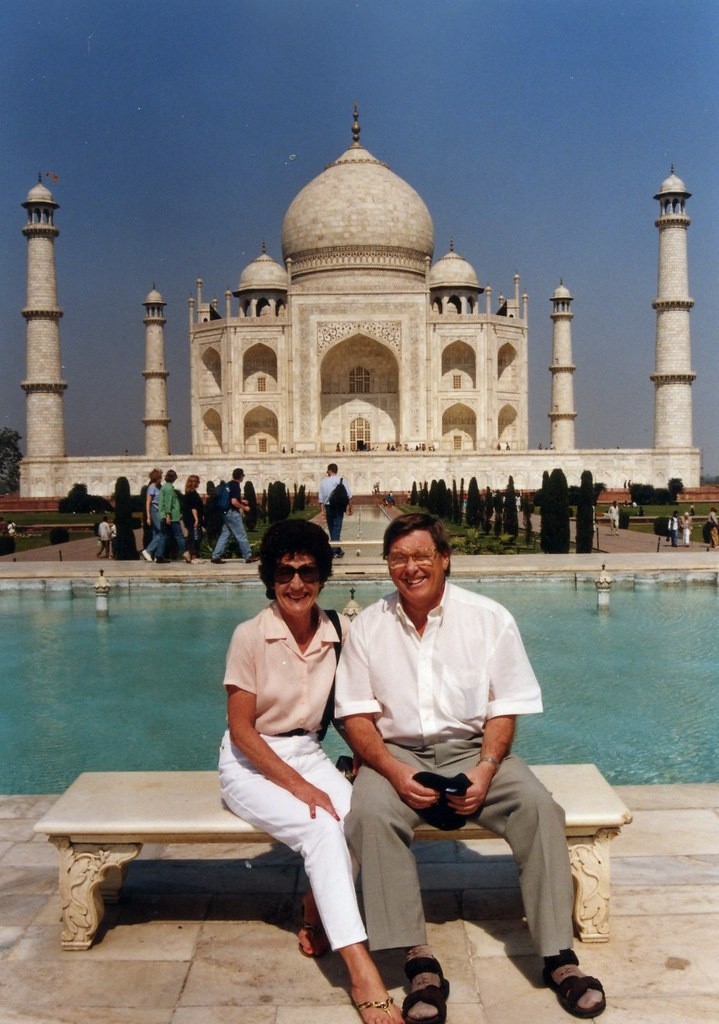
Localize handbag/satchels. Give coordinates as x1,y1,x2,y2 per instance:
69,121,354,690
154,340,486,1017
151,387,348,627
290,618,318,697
164,525,178,560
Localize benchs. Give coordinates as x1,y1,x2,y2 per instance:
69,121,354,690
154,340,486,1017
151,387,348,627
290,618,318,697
32,764,632,950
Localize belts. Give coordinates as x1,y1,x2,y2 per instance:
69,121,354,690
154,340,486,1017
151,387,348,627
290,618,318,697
274,728,309,737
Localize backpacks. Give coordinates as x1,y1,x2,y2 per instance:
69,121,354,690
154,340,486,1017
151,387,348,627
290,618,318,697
330,476,349,512
217,483,239,510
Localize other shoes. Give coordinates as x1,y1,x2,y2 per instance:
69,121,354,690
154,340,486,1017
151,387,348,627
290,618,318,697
245,553,261,563
142,549,153,562
211,557,225,563
331,549,345,558
191,558,206,564
156,555,170,563
182,551,191,563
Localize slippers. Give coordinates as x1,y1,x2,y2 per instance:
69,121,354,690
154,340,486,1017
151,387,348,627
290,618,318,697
349,987,406,1024
298,904,329,958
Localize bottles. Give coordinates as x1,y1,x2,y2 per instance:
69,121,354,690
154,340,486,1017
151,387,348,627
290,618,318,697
194,528,198,540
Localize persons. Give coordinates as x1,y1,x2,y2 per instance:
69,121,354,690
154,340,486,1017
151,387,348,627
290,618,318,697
679,512,694,546
628,479,631,487
216,519,408,1024
637,506,645,516
333,513,607,1024
336,441,345,451
708,505,719,548
506,442,510,449
386,442,409,451
608,500,619,535
384,491,395,507
0,515,16,535
464,493,467,507
97,467,260,564
497,443,501,450
550,442,554,449
666,510,682,547
407,491,411,503
623,500,638,508
318,463,353,555
623,479,627,488
689,503,695,515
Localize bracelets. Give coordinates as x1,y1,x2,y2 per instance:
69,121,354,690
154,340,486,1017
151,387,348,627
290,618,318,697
479,757,501,770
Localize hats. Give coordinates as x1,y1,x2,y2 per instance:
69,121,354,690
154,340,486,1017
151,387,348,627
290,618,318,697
407,772,474,831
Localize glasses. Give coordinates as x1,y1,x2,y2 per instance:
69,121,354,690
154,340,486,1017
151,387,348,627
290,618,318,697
273,563,320,584
385,548,438,570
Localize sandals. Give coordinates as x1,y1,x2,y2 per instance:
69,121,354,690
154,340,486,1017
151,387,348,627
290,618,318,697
543,947,606,1018
406,955,449,1024
